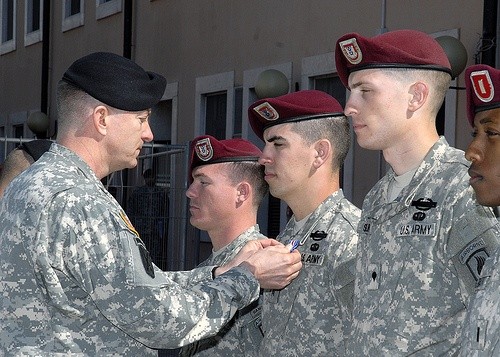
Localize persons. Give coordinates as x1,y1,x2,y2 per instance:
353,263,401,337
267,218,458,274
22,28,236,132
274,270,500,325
461,64,500,357
159,135,271,357
125,168,170,271
0,52,302,357
1,140,54,201
247,89,362,357
334,30,500,356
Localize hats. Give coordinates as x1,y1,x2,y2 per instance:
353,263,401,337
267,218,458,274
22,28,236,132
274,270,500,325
465,64,500,130
334,30,455,94
63,52,168,111
20,139,55,163
247,90,344,144
188,135,263,185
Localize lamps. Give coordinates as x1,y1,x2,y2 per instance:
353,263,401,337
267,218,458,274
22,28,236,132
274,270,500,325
28,112,49,139
255,69,288,99
433,36,467,90
